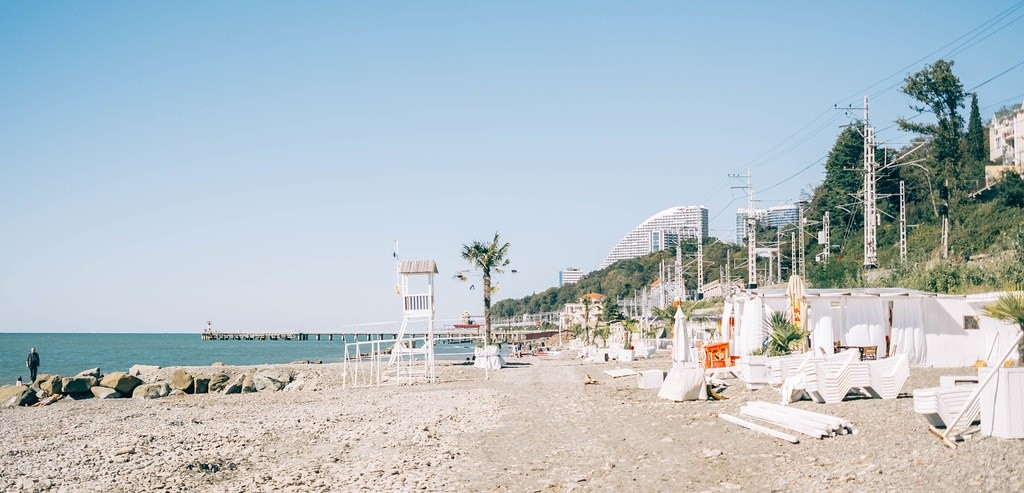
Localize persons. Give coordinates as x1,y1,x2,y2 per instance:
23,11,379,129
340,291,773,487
26,348,39,384
208,321,214,332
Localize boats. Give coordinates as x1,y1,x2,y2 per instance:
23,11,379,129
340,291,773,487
453,319,484,329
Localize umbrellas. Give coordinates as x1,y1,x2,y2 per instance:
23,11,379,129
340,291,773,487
673,305,689,362
787,275,809,351
